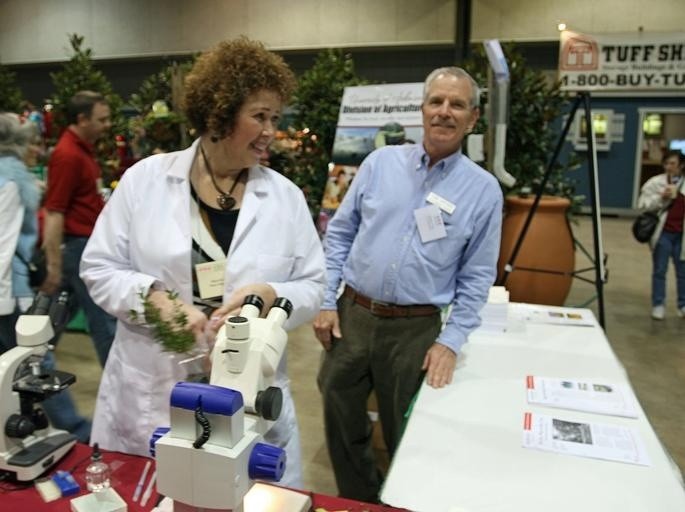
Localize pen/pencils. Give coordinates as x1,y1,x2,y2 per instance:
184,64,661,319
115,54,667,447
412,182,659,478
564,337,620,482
132,461,156,507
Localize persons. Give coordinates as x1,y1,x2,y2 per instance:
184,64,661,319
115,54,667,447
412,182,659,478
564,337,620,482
636,149,685,321
312,67,506,505
18,102,46,135
0,109,92,442
40,89,116,371
80,36,326,489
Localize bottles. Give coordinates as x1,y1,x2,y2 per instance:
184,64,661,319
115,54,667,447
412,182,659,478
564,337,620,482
85,443,110,494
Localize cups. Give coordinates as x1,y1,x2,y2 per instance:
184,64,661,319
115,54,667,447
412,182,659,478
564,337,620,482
666,184,676,199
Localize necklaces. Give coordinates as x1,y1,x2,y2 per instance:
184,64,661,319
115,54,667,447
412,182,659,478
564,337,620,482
199,143,244,211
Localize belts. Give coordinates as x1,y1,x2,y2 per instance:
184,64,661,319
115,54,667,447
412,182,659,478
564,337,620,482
342,283,442,317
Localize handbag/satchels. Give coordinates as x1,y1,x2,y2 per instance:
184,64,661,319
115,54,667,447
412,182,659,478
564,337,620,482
27,250,48,288
630,212,660,244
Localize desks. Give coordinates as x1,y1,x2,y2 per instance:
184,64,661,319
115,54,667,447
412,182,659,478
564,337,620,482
0,441,411,511
379,299,684,511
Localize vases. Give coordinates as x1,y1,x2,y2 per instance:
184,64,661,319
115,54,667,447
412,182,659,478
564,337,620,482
494,189,576,306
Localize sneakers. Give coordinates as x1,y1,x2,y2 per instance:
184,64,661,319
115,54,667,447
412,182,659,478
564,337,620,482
650,303,666,321
676,306,685,317
